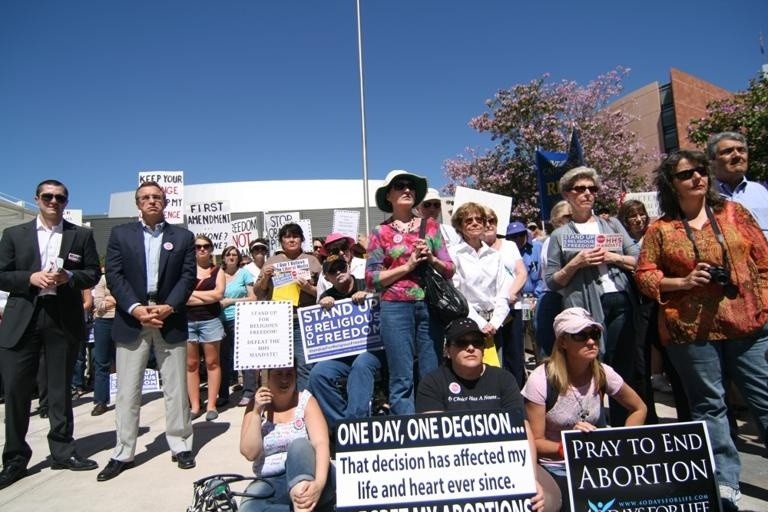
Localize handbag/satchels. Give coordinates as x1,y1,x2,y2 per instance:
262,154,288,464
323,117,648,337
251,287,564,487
186,472,275,512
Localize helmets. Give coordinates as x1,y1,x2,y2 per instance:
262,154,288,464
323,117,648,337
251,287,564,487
505,221,527,235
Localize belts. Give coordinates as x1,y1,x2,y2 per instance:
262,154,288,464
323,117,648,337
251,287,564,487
147,291,159,302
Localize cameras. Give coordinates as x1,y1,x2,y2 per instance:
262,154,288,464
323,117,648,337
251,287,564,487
704,265,729,286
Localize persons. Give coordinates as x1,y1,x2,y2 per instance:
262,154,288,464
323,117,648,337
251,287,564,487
98,183,195,482
241,352,335,506
541,202,583,291
506,223,545,300
445,204,510,374
242,240,269,286
317,232,366,303
181,238,224,419
474,208,527,377
416,320,562,511
706,132,767,240
366,170,453,413
546,167,639,373
308,255,387,417
617,201,691,425
1,179,96,489
254,224,314,393
597,206,609,221
521,308,646,505
92,265,116,416
69,289,92,407
637,149,767,507
417,187,461,252
218,247,257,405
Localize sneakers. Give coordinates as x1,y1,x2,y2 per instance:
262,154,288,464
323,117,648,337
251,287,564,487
652,376,672,393
718,483,742,511
239,396,253,406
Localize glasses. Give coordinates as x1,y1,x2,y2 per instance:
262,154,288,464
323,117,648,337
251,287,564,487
424,201,441,209
330,242,349,255
466,217,483,223
39,192,67,202
395,182,415,190
670,166,710,180
568,185,599,193
484,219,496,224
314,246,322,250
195,243,211,250
571,331,602,340
449,338,483,348
329,263,346,273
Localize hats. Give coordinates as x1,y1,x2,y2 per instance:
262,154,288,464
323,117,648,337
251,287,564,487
373,169,428,212
322,254,347,274
319,233,355,255
553,305,605,335
445,318,487,344
249,241,269,250
422,186,442,203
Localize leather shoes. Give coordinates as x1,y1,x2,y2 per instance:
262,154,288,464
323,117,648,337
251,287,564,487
96,458,135,481
0,464,29,489
172,450,197,469
205,409,218,421
90,402,107,416
50,449,99,471
190,408,202,420
